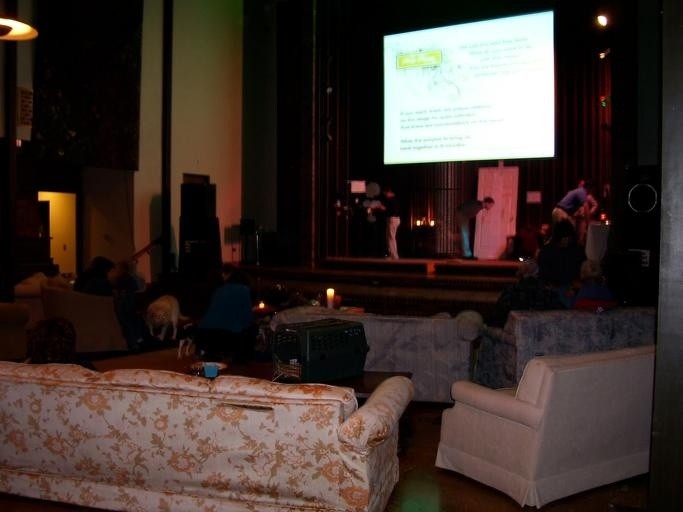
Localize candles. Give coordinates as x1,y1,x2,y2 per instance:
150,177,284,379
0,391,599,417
325,289,336,305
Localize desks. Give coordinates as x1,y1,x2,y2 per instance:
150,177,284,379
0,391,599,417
271,371,412,402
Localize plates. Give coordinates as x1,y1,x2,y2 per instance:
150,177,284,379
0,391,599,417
190,361,227,371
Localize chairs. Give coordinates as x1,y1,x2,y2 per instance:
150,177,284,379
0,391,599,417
40,271,128,357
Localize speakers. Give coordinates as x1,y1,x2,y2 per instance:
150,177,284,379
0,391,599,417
29,0,144,172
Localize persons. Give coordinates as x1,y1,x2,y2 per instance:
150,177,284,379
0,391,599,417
496,244,573,325
551,180,595,249
455,196,496,260
22,316,97,372
576,175,599,245
115,261,148,343
370,188,403,259
74,255,144,350
553,219,585,260
195,272,260,363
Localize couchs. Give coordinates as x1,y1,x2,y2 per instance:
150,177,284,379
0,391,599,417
475,308,656,390
1,365,416,512
435,348,655,512
272,306,481,403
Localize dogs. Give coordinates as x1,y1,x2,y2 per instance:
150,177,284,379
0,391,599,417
146,294,191,342
